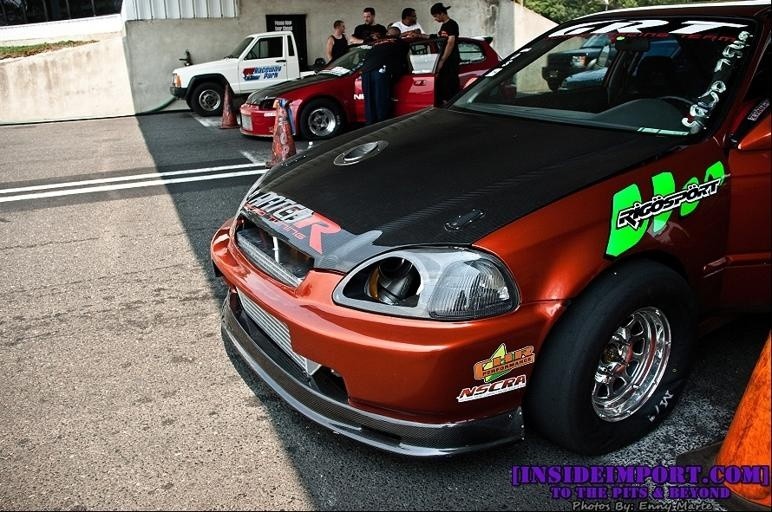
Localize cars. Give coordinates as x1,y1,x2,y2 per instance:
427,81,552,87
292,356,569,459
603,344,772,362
238,36,505,142
201,1,772,462
556,41,680,98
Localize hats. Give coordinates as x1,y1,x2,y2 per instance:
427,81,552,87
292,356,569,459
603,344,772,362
431,3,451,15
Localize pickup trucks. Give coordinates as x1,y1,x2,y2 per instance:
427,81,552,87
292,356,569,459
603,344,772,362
171,31,340,119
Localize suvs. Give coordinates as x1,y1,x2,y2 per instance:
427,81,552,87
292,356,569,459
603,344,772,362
541,30,623,93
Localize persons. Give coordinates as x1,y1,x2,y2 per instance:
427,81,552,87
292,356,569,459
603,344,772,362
327,20,364,62
352,7,425,125
430,3,461,107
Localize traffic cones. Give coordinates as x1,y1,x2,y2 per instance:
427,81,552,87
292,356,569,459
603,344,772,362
669,327,770,512
261,95,306,168
218,84,242,131
